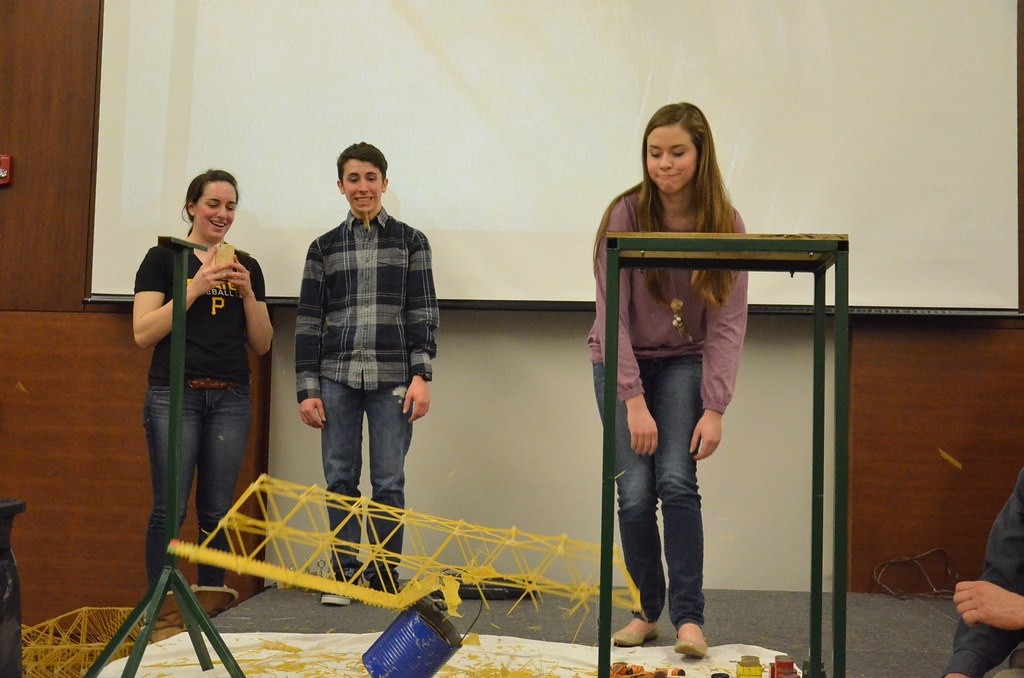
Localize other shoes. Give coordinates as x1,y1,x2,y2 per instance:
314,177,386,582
672,628,708,656
319,576,359,606
612,610,660,646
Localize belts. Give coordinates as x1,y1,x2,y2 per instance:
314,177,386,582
187,377,239,389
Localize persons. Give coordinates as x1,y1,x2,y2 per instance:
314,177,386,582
133,170,275,642
941,468,1024,678
589,100,749,659
296,141,441,607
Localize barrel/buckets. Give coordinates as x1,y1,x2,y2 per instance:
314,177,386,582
362,597,462,678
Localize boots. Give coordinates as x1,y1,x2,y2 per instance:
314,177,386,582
149,590,183,643
190,582,239,624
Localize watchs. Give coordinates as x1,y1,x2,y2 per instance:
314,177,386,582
416,372,433,382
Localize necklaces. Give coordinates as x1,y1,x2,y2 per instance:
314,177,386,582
665,266,691,339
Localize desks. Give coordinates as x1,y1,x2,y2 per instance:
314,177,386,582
597,229,851,678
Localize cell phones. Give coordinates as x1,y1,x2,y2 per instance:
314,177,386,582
214,244,234,281
